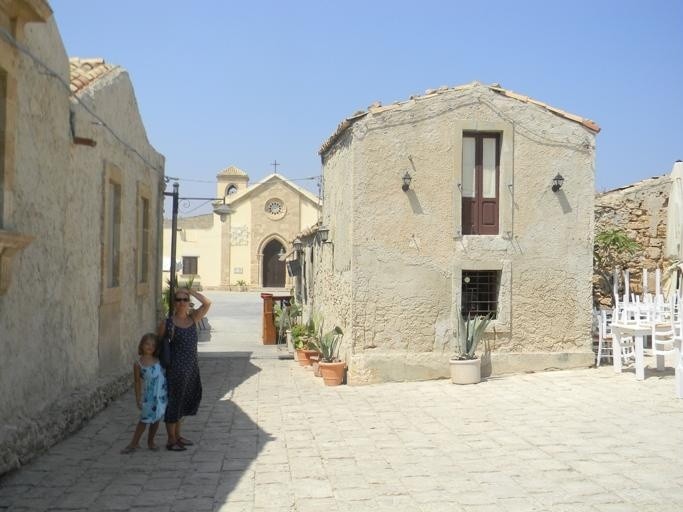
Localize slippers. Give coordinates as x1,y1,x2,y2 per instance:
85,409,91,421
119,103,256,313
120,444,159,455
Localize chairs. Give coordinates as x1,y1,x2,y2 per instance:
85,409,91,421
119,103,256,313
593,268,683,399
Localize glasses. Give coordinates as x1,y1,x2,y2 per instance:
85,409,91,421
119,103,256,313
175,297,189,303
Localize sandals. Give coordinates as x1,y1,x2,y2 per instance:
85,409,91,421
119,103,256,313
178,436,195,447
165,441,188,452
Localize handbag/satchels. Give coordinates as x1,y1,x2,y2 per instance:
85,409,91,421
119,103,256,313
158,318,171,370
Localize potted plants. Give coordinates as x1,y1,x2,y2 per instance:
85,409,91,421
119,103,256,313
450,308,494,385
273,300,346,386
234,280,248,292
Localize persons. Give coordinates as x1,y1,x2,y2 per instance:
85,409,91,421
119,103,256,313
119,332,168,454
155,286,212,450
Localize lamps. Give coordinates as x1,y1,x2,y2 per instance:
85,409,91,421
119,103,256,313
552,172,565,192
317,226,331,245
401,171,412,191
